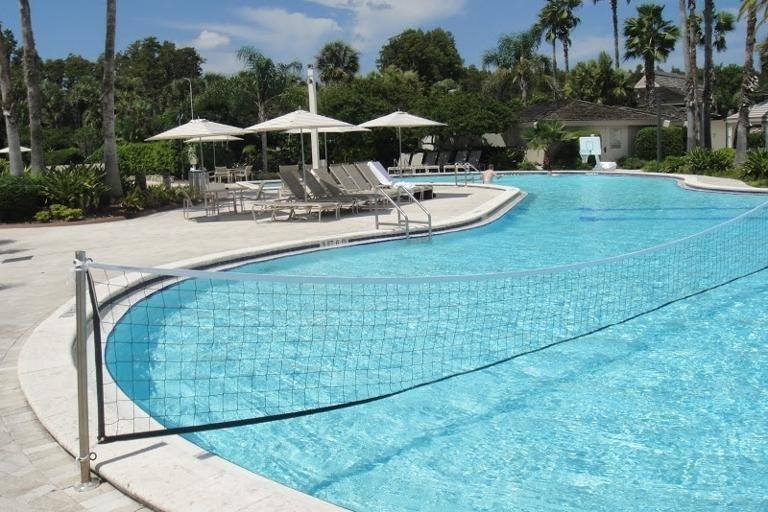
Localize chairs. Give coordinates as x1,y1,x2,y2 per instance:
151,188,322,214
180,150,483,222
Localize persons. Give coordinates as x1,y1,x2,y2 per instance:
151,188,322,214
482,163,500,184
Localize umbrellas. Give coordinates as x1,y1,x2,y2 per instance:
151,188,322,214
357,108,448,180
182,134,245,169
143,115,257,172
1,145,33,155
243,104,372,199
278,125,370,174
